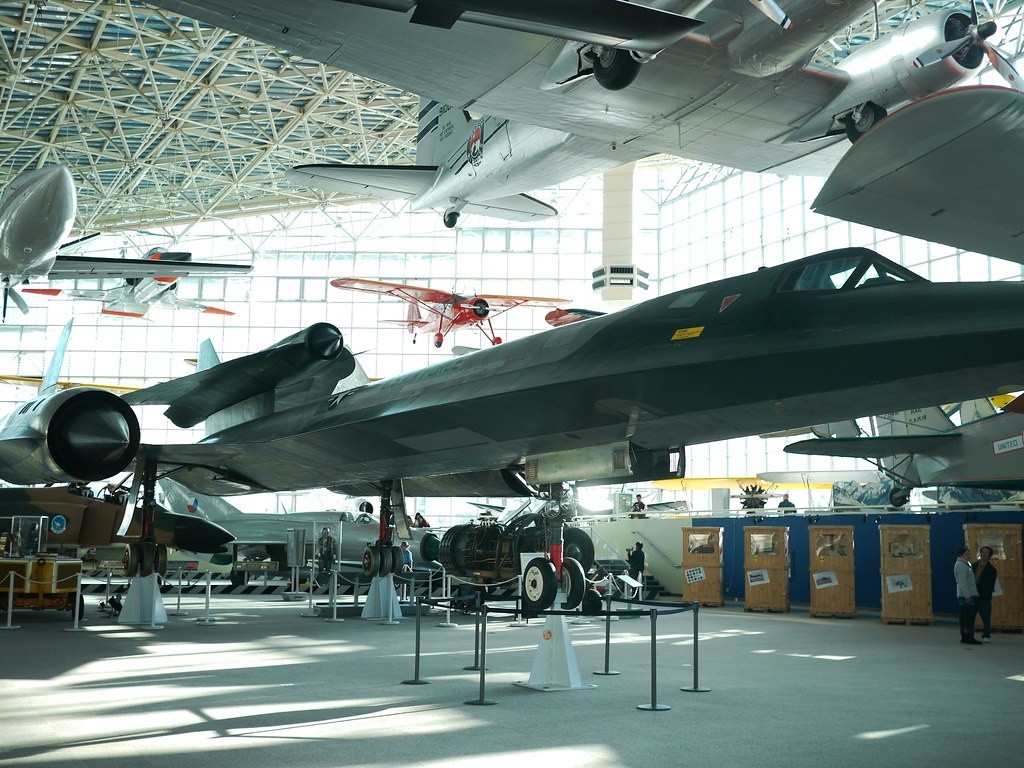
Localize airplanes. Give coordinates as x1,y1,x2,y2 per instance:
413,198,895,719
142,0,1022,227
20,246,238,319
1,163,255,322
0,248,1024,612
332,274,571,348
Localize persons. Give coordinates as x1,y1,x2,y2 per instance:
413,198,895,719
314,512,432,580
113,595,125,610
626,492,797,601
955,547,998,647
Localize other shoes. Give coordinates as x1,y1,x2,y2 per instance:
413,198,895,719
984,637,991,643
960,637,983,644
